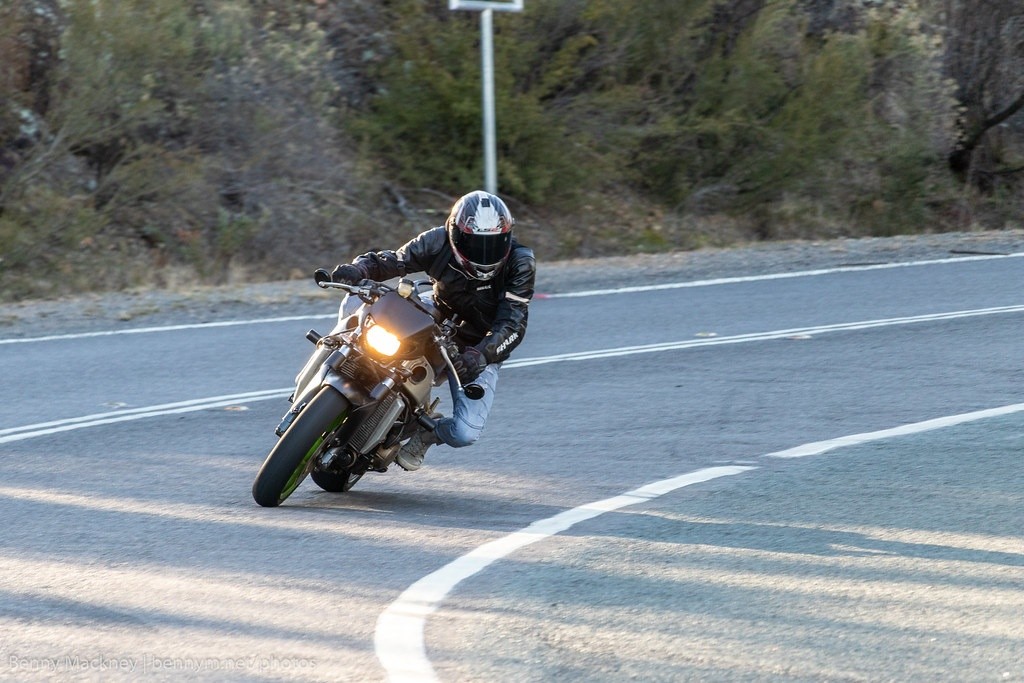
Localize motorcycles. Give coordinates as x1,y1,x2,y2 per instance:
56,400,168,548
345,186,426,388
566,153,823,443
250,267,488,510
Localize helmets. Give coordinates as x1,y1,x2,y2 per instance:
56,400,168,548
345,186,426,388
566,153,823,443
444,190,515,281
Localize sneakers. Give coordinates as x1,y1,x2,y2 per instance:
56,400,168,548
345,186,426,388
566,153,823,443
397,430,431,473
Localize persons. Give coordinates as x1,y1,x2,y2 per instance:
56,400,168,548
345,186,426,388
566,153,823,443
329,189,538,470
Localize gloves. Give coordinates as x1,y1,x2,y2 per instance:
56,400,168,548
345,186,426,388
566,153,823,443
450,348,488,387
330,263,364,287
